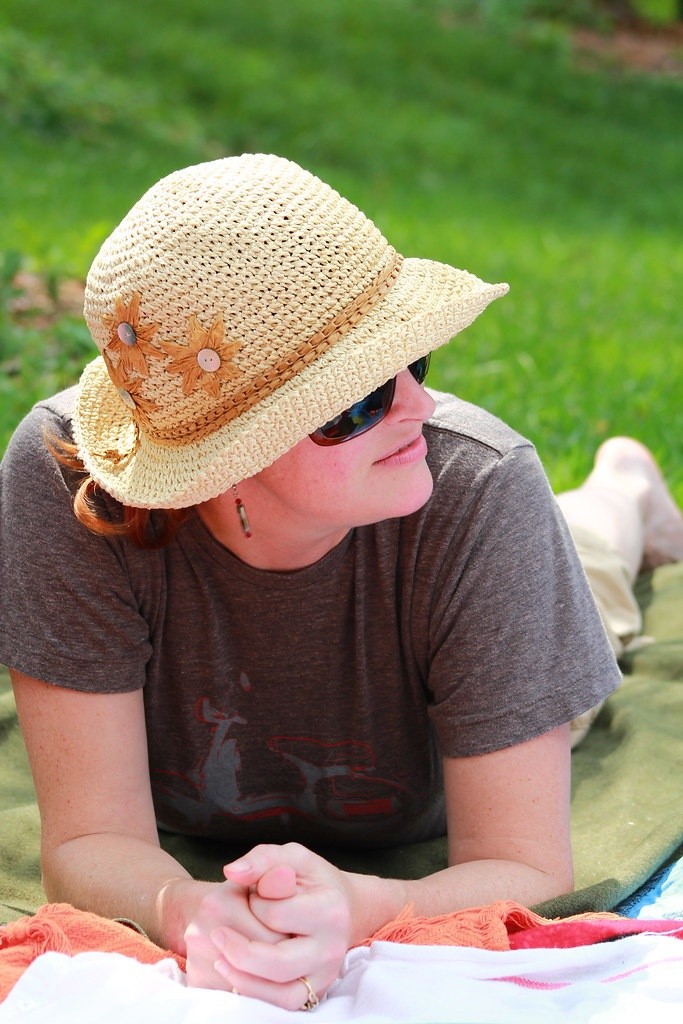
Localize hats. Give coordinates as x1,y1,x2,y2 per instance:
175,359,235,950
71,149,507,502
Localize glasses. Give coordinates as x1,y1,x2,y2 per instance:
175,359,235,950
307,352,435,447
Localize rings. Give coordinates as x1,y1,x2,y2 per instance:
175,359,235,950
232,988,241,996
299,977,319,1012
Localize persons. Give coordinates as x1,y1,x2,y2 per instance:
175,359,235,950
1,155,683,1014
